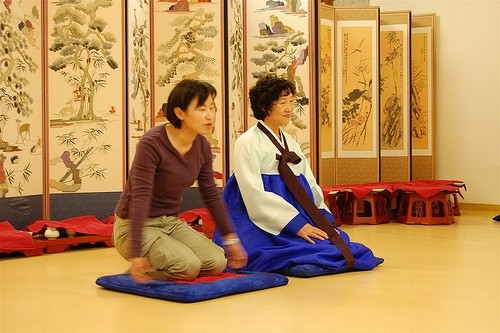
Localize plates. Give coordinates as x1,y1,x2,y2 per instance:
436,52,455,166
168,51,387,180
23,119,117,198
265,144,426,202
454,183,464,186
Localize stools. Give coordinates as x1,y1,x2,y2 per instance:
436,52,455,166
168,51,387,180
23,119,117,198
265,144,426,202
323,186,463,227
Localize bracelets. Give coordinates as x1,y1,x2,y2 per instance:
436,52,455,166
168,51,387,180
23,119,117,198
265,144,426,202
222,238,241,246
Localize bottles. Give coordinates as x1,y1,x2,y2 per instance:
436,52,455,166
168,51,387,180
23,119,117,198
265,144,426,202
44,227,60,240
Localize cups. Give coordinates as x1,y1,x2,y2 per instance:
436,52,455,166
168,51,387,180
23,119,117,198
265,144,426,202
65,228,76,238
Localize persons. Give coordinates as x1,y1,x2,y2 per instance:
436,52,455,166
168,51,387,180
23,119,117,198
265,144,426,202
214,75,376,272
111,79,247,283
